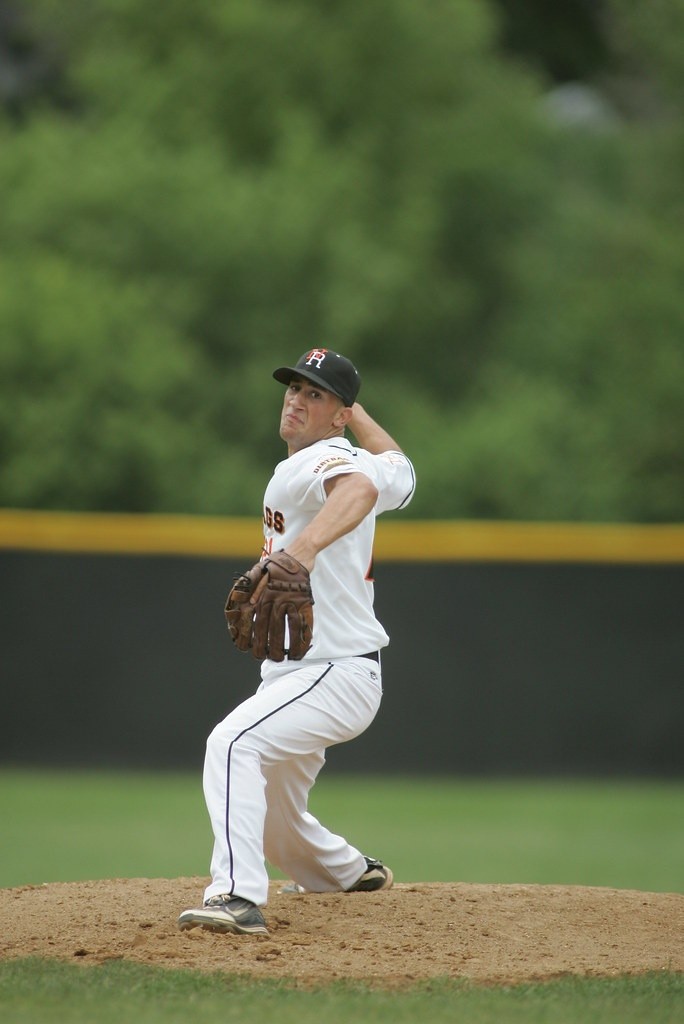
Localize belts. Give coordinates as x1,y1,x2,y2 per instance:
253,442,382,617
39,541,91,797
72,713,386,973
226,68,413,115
354,650,379,662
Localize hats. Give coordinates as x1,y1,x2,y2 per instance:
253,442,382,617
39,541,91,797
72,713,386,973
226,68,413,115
272,349,361,408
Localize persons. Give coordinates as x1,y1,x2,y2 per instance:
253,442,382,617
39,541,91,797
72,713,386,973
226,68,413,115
179,348,417,935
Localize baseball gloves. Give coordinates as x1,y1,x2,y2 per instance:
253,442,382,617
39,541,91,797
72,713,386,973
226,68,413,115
217,549,315,665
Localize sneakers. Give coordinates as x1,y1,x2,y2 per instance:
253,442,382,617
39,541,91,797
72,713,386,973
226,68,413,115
282,856,394,894
178,895,270,936
